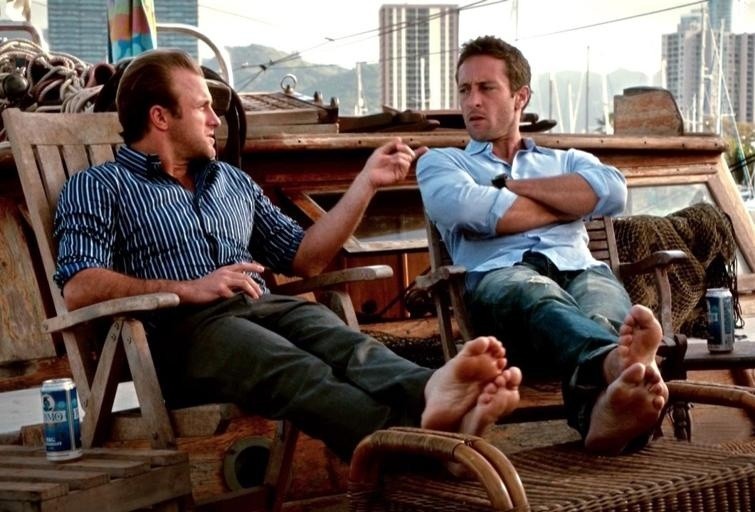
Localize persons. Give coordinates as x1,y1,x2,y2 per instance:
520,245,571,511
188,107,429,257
415,36,668,454
54,49,522,467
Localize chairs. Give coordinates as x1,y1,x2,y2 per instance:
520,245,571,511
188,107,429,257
0,109,753,509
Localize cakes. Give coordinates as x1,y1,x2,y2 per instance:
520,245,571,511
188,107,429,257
40,378,83,461
706,287,735,352
